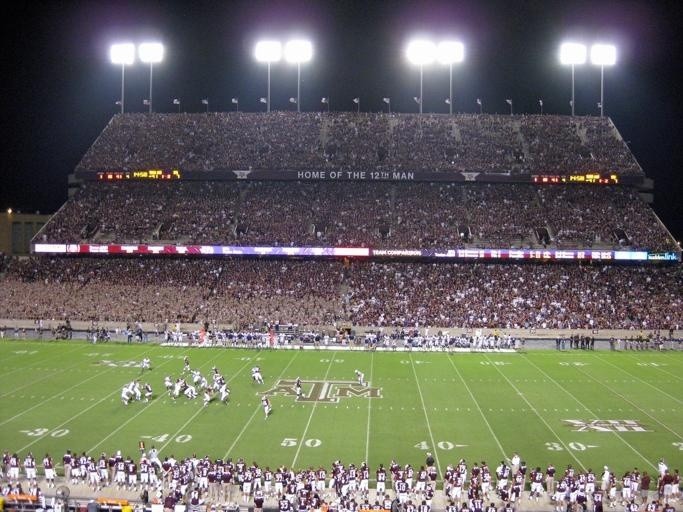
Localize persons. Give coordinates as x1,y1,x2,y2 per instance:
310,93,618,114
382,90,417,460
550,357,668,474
355,370,365,384
252,365,264,383
262,395,272,419
122,354,231,406
294,377,305,402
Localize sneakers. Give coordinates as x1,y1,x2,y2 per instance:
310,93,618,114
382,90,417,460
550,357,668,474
71,479,160,493
517,497,679,508
8,482,55,489
